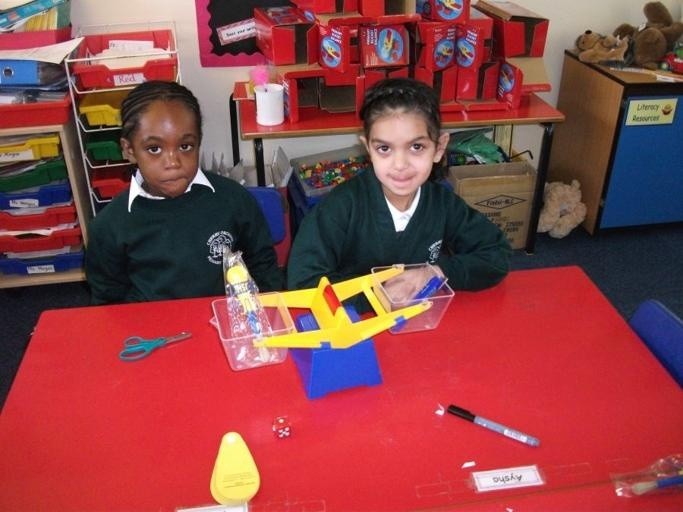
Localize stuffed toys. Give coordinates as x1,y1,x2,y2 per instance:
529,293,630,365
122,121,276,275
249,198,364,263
573,26,629,65
610,0,680,71
534,177,588,241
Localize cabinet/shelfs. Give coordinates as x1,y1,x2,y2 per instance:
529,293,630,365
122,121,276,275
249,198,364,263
64,22,183,218
1,123,88,289
548,48,682,240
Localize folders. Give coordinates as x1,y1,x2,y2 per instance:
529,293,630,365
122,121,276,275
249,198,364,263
0,59,66,85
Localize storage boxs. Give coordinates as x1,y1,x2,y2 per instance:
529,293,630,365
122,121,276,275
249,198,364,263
446,161,536,250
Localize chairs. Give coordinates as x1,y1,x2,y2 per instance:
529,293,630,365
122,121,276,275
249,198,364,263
628,298,681,388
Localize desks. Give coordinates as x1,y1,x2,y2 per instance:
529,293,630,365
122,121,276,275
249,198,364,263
0,264,683,511
228,81,567,256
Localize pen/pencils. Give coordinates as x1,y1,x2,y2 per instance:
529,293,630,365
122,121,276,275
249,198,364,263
446,405,540,447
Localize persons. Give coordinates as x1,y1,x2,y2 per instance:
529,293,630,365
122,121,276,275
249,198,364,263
66,76,287,307
276,72,514,308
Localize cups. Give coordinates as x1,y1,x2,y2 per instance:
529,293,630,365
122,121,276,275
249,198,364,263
253,83,286,126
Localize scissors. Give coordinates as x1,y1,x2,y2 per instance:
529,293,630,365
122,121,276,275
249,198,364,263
118,334,192,362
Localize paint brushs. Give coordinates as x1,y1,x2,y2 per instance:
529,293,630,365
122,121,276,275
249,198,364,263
631,475,683,496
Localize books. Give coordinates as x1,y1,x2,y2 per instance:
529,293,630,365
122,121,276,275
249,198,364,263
0,0,84,260
